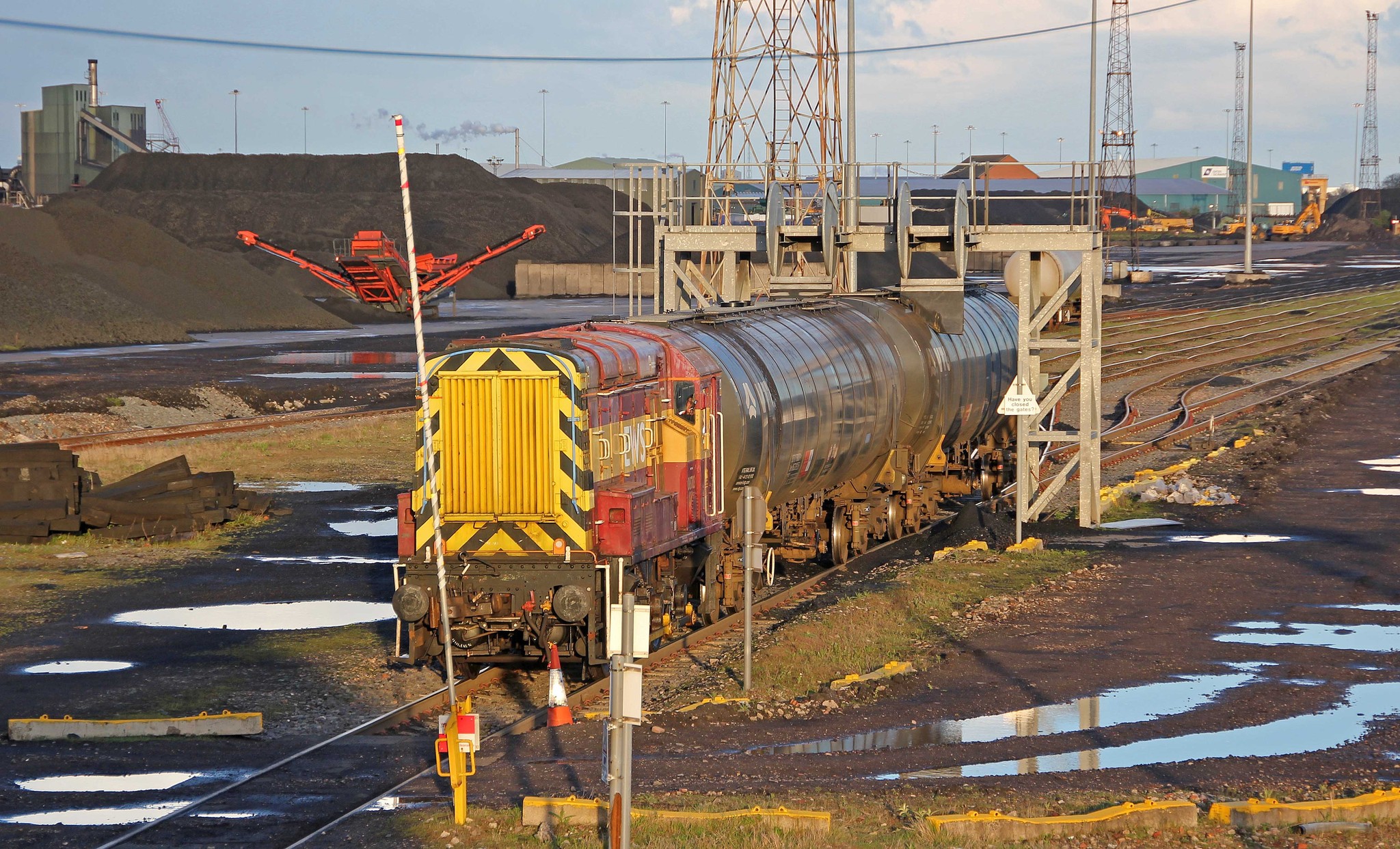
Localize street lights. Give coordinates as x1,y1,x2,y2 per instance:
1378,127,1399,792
537,88,549,167
1267,148,1274,167
903,140,913,178
998,131,1009,154
1056,137,1065,168
463,147,471,159
1222,108,1234,190
964,125,978,179
870,132,883,180
1192,146,1202,156
300,106,311,154
226,88,241,154
485,156,504,177
660,100,672,162
930,123,942,179
1151,143,1159,158
1352,102,1365,191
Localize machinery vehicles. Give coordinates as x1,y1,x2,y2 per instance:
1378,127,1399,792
1220,215,1267,243
1266,201,1321,241
1100,205,1196,235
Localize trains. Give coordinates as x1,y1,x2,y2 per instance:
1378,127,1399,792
384,282,1033,682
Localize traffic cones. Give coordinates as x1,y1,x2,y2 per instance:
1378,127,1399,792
545,642,576,729
